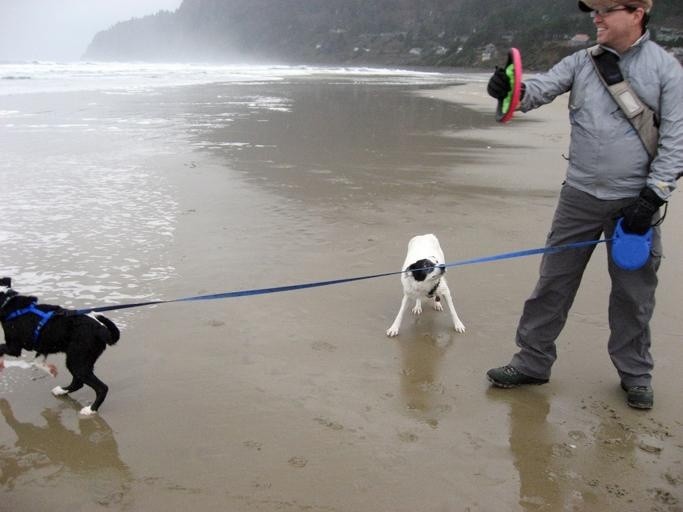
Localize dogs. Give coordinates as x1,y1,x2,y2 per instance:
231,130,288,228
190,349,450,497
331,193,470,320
385,233,467,337
0,277,120,416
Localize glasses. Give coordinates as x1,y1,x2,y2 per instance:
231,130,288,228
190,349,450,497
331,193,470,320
588,8,631,19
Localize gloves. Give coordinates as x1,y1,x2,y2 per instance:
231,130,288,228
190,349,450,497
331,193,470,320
485,66,525,104
609,185,669,237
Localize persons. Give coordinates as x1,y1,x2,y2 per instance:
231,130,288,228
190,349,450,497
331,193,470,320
483,1,681,409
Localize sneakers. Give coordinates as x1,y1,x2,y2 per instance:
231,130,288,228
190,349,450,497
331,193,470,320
484,362,549,390
619,379,654,410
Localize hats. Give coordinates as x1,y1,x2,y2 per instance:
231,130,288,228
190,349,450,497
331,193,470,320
576,0,654,19
